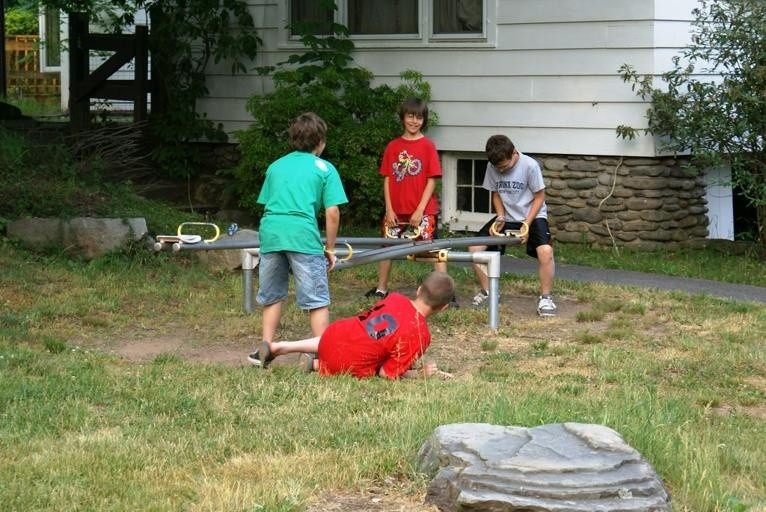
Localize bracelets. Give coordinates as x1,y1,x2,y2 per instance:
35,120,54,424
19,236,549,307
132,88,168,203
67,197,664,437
322,245,338,255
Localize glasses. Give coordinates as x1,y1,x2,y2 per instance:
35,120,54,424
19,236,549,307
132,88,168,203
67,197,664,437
490,154,513,170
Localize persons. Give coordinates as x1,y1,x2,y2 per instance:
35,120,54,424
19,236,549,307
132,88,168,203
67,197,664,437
363,98,462,311
466,134,557,319
246,111,351,373
245,269,457,382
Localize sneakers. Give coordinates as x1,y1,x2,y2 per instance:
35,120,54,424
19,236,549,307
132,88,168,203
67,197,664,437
536,293,557,318
470,288,501,310
449,296,460,309
362,287,389,299
298,352,316,373
247,341,276,369
